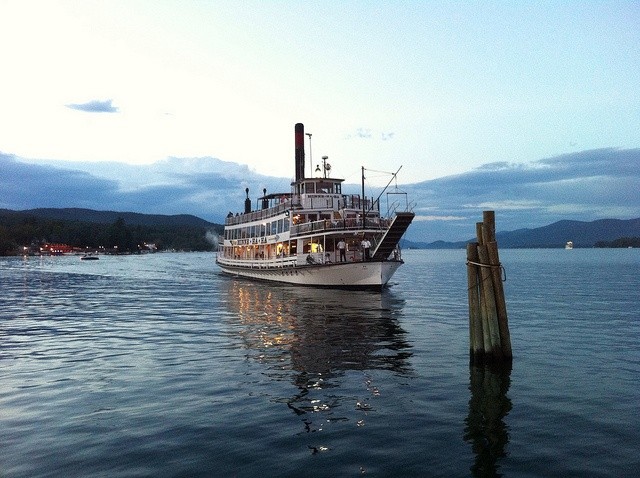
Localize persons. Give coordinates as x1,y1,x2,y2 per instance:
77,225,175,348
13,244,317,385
227,209,262,225
260,251,264,257
336,238,346,261
361,237,371,260
279,251,285,256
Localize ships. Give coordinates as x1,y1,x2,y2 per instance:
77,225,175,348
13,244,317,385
222,277,418,455
215,122,416,290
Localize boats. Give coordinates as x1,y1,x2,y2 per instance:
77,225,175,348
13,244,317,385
80,255,100,261
